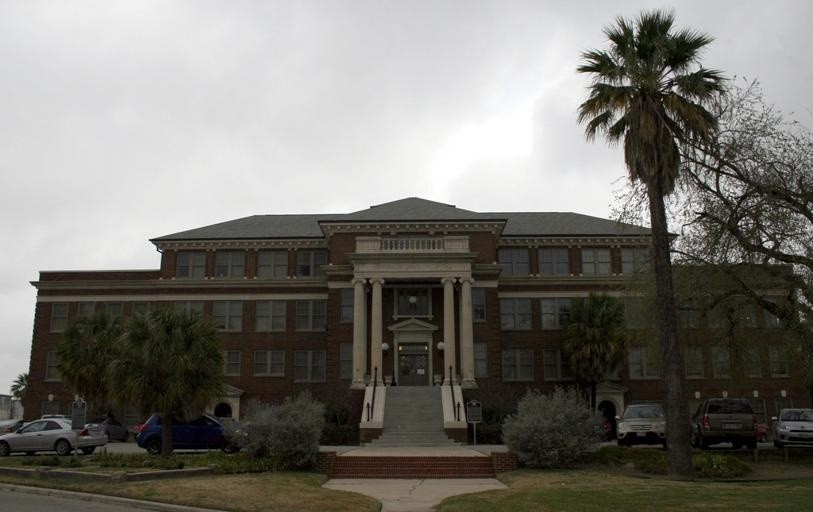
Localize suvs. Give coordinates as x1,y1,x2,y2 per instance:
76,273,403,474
688,397,757,451
614,403,666,452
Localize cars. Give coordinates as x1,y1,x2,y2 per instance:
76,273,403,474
134,411,245,454
41,414,72,420
755,422,768,444
770,408,812,448
0,418,108,457
85,417,129,442
